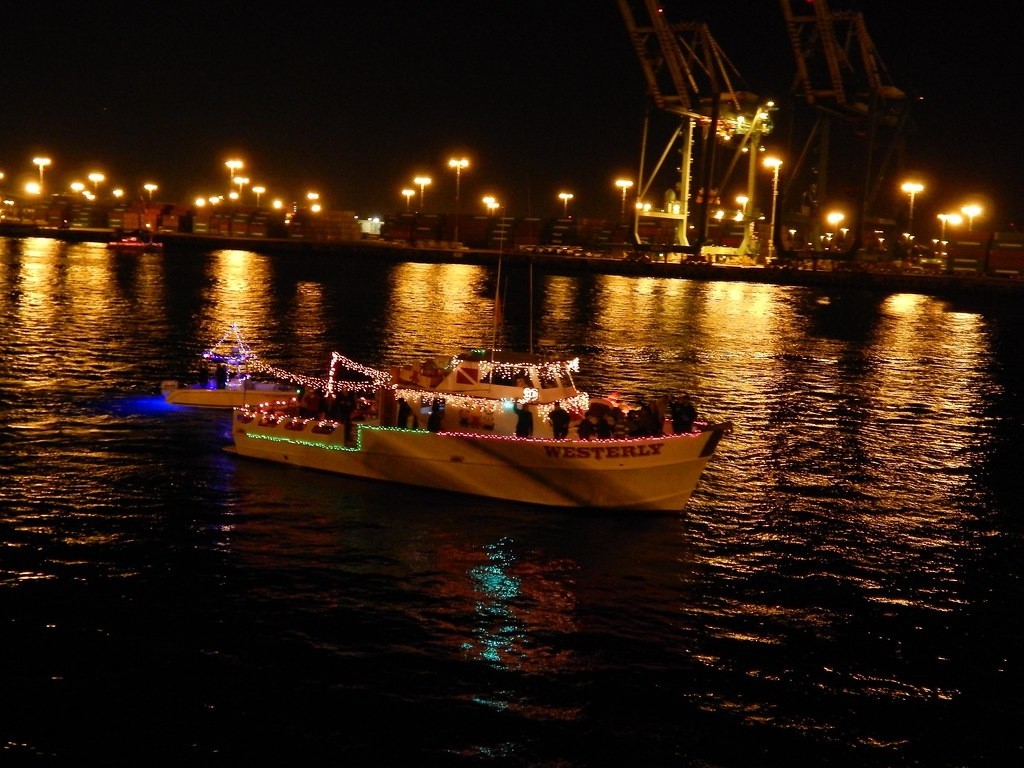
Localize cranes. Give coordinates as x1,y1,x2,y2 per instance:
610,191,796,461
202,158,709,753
620,0,787,269
772,0,913,266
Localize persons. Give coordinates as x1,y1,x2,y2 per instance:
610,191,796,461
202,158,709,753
548,402,568,439
297,385,350,421
598,412,611,438
215,363,227,389
428,405,441,433
577,412,596,440
397,397,413,429
514,397,533,438
627,394,668,437
669,395,697,433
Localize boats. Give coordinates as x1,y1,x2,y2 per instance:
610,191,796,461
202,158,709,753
156,171,735,521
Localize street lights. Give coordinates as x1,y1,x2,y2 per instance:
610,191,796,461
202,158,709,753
614,177,634,225
448,156,470,243
558,191,574,219
225,158,244,190
900,179,925,236
961,201,987,232
33,156,53,200
252,186,266,208
